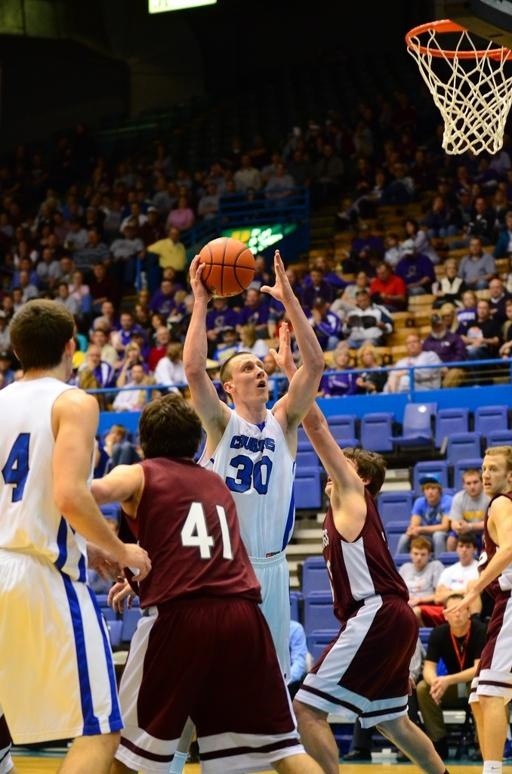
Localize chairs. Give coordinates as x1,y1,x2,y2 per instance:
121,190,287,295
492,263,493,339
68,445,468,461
414,461,447,497
446,432,480,466
377,492,412,531
454,459,483,494
295,452,323,476
326,416,360,449
297,425,315,450
295,470,321,508
387,403,438,445
454,704,475,759
304,591,343,633
308,629,341,663
389,534,410,567
475,406,508,438
290,592,299,622
436,410,469,447
303,557,331,597
487,429,512,450
420,628,433,641
442,552,460,562
360,413,394,451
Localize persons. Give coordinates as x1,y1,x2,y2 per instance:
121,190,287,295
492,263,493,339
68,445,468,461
170,249,326,774
66,323,119,408
0,142,199,335
397,473,452,560
1,300,152,774
396,537,446,627
205,288,271,364
341,636,428,764
448,469,491,534
443,445,512,774
346,341,390,393
379,336,446,392
198,84,512,250
436,531,481,595
89,396,324,774
311,220,512,348
416,595,486,761
269,321,452,773
282,620,305,699
0,322,26,392
252,254,335,319
318,347,355,398
93,422,125,478
103,515,122,538
112,325,192,413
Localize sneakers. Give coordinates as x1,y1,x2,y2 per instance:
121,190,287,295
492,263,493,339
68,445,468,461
435,742,448,761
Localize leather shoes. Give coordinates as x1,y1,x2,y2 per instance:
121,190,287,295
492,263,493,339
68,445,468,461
343,750,372,761
396,754,410,762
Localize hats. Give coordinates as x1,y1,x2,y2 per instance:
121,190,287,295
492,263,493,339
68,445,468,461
313,295,326,307
221,326,235,332
418,473,442,486
401,239,416,255
430,313,443,325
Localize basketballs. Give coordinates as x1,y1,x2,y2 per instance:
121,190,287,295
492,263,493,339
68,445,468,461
197,237,256,297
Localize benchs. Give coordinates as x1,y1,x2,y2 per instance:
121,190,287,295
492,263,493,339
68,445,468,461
313,203,510,380
